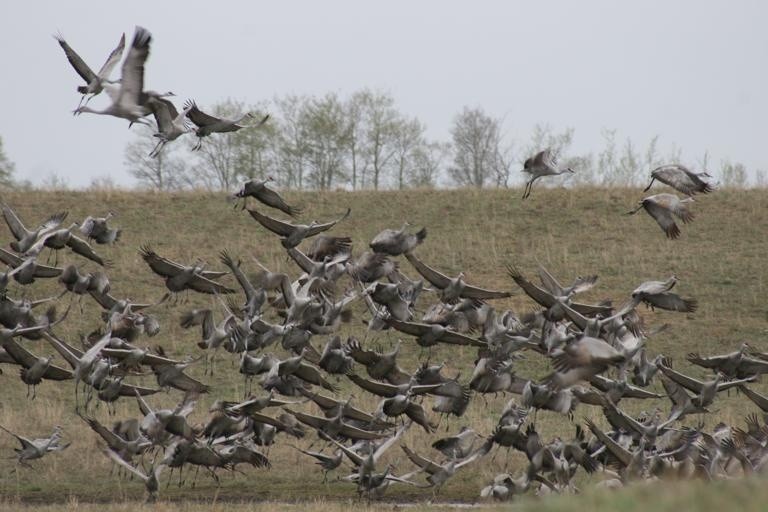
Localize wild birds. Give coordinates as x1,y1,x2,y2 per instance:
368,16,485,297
233,176,305,221
643,163,711,197
623,193,696,238
516,145,578,199
54,24,270,160
0,207,768,501
